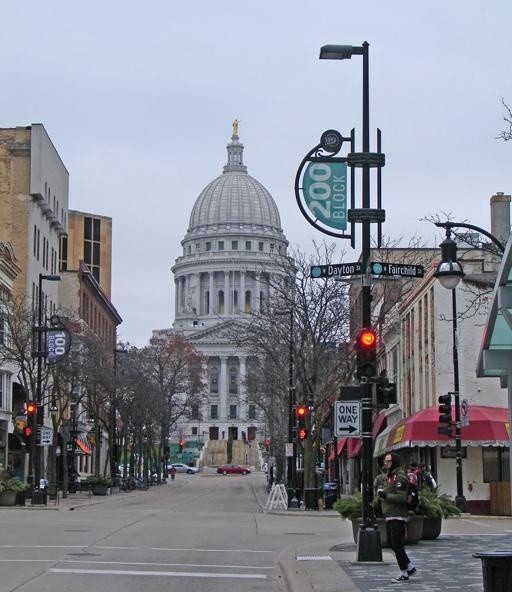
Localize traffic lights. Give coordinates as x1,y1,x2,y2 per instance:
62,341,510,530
358,331,376,378
27,402,37,429
437,393,453,437
296,406,307,442
179,439,183,449
23,427,33,453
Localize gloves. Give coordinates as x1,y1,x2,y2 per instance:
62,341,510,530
378,491,386,499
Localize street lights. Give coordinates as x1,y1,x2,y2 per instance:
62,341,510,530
110,347,127,489
320,34,384,563
273,308,294,487
32,274,62,504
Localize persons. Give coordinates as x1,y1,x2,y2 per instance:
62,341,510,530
189,461,194,467
373,462,437,495
68,464,80,477
170,465,176,480
379,451,418,583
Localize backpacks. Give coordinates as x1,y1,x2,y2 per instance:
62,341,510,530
407,469,419,485
396,473,419,511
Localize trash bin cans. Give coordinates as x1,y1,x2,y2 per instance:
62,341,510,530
324,483,340,511
32,488,48,506
471,552,511,592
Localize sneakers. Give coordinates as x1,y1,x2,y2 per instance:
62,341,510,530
392,568,416,582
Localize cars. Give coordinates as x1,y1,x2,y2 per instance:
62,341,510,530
216,462,251,475
165,463,200,474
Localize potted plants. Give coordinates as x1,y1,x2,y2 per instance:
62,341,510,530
333,478,466,548
87,473,115,495
0,474,25,506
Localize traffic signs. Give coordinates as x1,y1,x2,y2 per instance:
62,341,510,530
335,401,362,438
41,429,54,446
